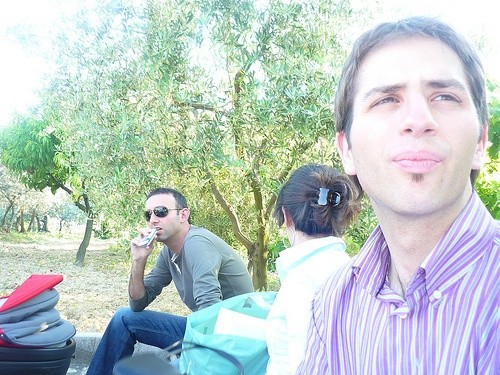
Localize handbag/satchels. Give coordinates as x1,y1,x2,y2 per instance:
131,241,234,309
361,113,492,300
178,291,278,375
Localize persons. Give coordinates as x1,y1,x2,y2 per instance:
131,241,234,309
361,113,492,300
86,188,255,375
261,163,362,375
294,15,500,375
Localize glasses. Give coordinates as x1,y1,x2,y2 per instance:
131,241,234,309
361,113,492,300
144,206,183,222
